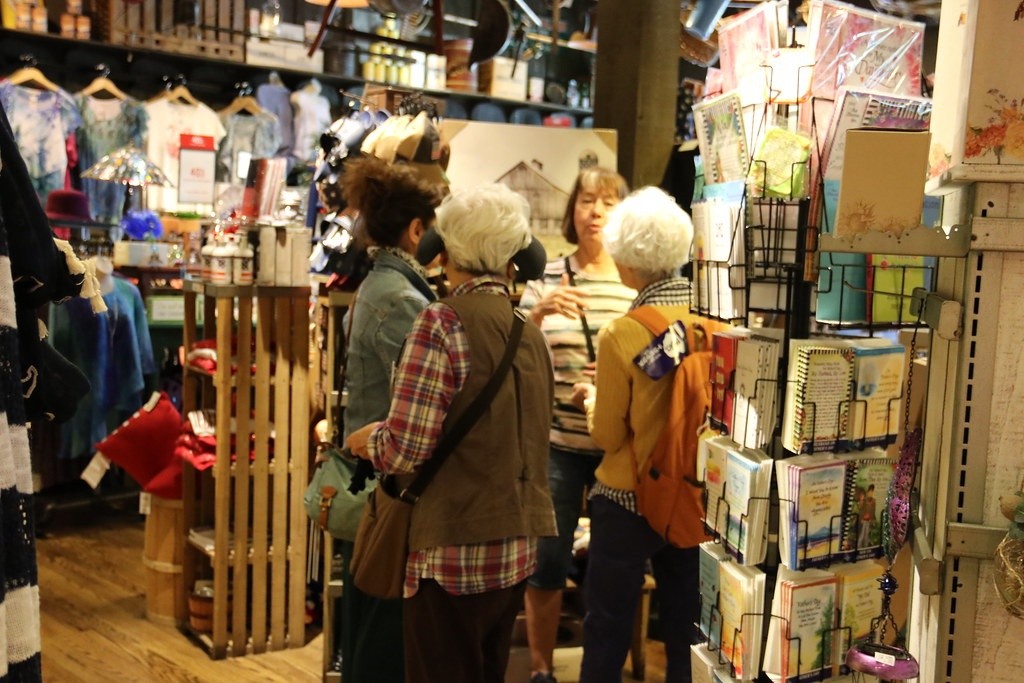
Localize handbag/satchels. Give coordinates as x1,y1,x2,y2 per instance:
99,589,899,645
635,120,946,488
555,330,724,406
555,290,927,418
345,478,411,604
304,442,382,542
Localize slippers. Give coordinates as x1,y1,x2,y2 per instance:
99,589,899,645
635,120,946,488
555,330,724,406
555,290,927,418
306,103,398,293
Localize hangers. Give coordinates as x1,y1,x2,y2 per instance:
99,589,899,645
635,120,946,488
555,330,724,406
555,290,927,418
0,53,285,127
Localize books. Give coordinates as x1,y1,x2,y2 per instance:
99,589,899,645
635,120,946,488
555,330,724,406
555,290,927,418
683,0,934,683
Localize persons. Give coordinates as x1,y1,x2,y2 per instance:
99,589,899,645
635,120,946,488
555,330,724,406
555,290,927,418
517,164,633,682
565,185,736,683
339,156,449,683
341,180,559,683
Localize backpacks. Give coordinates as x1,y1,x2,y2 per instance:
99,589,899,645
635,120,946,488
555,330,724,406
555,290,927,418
620,304,726,548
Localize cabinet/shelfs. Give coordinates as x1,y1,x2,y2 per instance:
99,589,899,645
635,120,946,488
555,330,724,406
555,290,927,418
672,79,970,683
179,220,313,661
